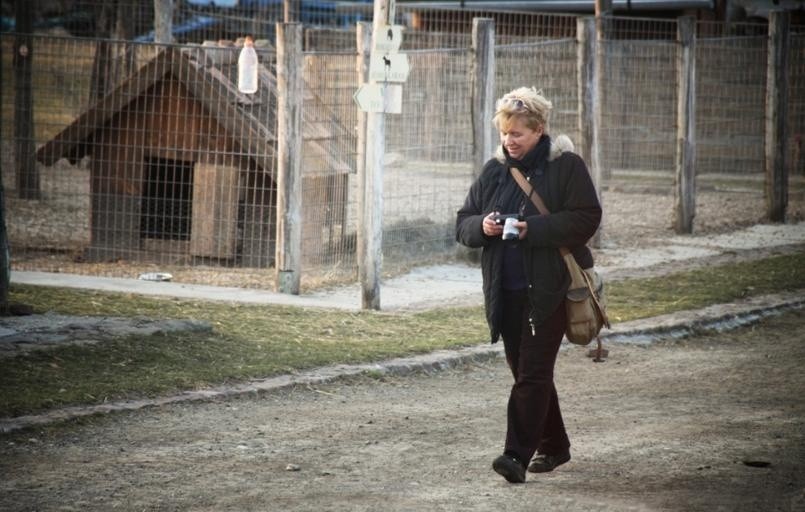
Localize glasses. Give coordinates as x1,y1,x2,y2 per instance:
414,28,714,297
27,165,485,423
504,98,532,112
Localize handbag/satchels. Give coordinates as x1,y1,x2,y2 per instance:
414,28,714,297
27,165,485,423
565,255,610,345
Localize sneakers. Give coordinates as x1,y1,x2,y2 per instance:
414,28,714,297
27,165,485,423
493,451,570,483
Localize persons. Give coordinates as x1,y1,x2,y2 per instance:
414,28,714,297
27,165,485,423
453,84,602,485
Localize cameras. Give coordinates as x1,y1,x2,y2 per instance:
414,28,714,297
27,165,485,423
492,213,523,245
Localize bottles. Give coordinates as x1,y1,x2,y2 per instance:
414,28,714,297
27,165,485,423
237,34,260,95
